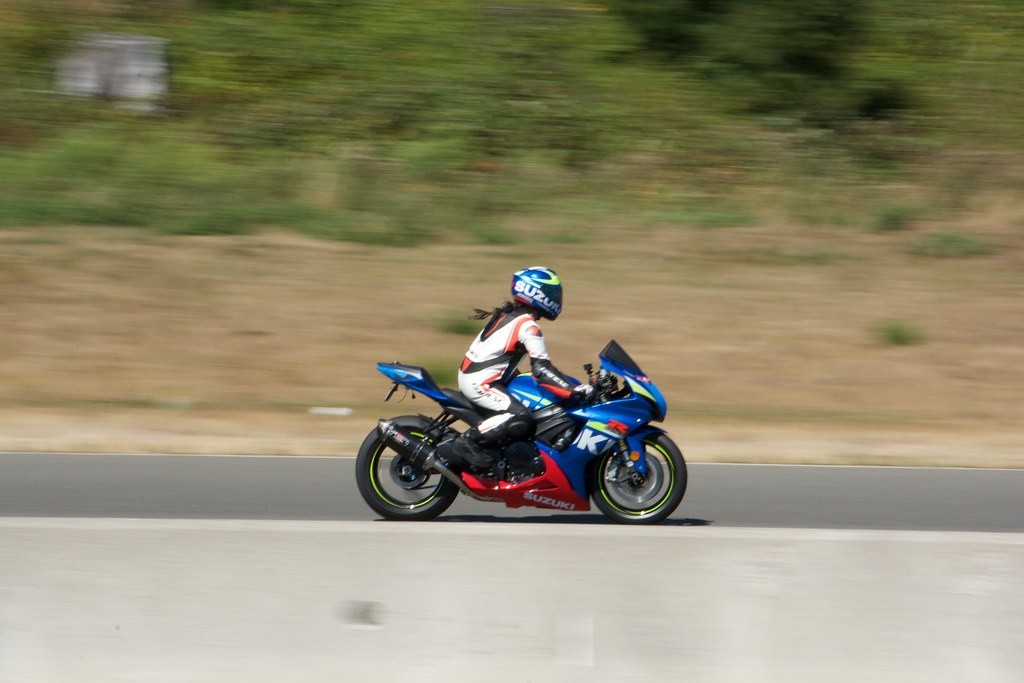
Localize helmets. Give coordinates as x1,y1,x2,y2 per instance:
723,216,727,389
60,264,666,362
511,266,562,320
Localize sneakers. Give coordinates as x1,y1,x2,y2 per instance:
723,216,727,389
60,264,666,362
453,426,496,468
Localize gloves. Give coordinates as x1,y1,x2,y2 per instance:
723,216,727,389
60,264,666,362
592,368,610,382
573,384,597,402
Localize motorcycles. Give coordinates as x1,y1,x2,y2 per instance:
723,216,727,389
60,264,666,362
354,338,689,526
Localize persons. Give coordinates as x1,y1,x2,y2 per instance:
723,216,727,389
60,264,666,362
453,266,593,467
